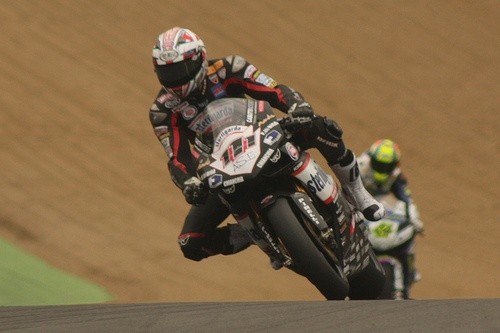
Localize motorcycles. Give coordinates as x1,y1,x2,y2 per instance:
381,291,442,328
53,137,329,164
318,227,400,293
184,96,389,300
366,195,426,303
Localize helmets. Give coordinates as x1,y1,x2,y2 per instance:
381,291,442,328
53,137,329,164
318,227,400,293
371,139,402,183
148,27,208,99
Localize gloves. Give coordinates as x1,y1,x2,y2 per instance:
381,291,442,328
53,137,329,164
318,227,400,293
288,102,317,127
181,176,210,207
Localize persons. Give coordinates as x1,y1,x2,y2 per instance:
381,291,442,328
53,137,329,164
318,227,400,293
148,27,385,270
357,139,425,280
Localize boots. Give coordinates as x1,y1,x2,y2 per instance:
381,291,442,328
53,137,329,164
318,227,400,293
227,224,283,270
330,153,386,222
